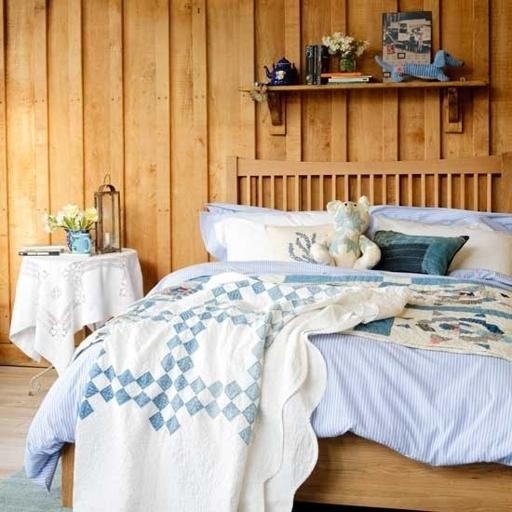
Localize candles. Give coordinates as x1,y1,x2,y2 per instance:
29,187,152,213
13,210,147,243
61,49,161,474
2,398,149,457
104,231,110,247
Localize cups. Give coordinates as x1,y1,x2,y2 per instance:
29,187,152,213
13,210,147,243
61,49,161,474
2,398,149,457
71,234,92,253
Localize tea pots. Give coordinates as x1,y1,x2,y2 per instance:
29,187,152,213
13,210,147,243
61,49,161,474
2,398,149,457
263,56,292,86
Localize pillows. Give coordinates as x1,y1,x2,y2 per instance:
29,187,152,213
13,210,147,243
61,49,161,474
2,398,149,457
211,209,335,269
370,227,471,276
368,214,511,277
222,216,334,264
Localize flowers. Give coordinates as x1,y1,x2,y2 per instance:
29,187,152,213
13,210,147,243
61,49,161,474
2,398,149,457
321,30,370,58
43,203,99,238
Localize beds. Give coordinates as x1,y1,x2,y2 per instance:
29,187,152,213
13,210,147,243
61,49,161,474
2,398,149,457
58,155,512,511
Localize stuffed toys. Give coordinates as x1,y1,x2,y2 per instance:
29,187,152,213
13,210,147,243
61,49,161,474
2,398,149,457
310,195,381,270
374,50,464,82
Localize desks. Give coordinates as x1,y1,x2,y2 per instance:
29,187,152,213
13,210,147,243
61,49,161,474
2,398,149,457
12,244,143,395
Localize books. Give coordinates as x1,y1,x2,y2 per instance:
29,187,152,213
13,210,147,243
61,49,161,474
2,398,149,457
18,246,64,256
321,72,373,83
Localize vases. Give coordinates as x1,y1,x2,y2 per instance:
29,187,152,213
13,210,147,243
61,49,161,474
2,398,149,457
67,234,91,254
339,59,357,72
